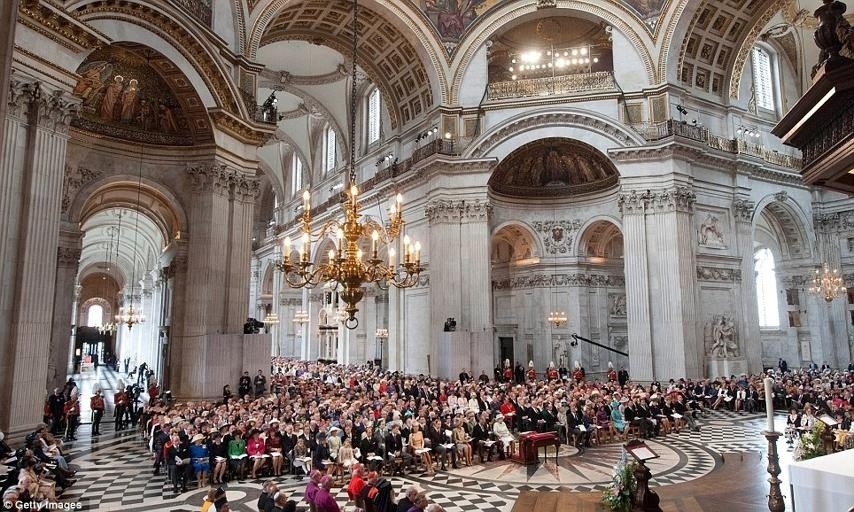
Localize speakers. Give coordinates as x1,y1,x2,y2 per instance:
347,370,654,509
444,320,456,332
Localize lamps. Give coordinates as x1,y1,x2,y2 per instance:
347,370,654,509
804,189,848,302
546,257,568,327
280,0,429,329
114,160,146,332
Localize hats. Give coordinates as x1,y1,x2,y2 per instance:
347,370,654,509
5,421,46,512
144,359,854,493
42,380,129,400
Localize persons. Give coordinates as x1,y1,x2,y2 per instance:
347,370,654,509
3,351,854,512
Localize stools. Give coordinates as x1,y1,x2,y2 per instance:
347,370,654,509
523,432,559,468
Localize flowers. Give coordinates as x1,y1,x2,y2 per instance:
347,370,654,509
602,461,637,511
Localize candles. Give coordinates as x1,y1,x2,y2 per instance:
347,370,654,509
763,378,776,433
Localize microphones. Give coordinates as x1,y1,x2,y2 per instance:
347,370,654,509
571,342,578,346
